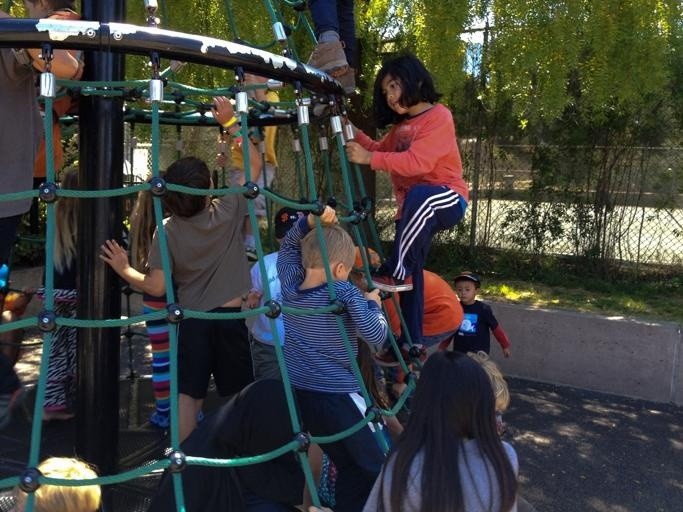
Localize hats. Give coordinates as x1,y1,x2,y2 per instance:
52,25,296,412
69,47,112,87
274,207,309,241
348,244,381,274
454,271,481,288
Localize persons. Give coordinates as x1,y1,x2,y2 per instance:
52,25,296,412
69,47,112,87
0,456,99,511
437,271,511,359
302,0,357,97
0,13,79,346
22,0,91,187
339,51,469,400
225,70,284,258
384,351,511,444
33,162,81,419
346,245,464,358
363,350,522,510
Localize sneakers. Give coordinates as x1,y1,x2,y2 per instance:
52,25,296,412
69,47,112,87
360,271,414,294
374,344,431,368
312,66,357,117
244,247,258,262
147,410,170,427
304,39,349,79
